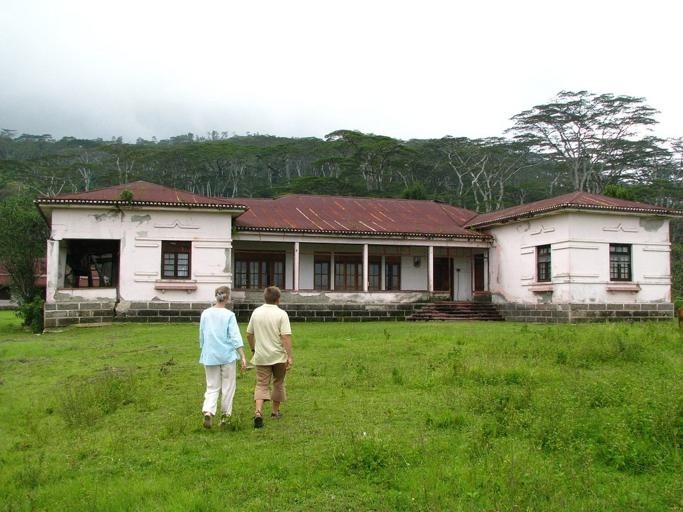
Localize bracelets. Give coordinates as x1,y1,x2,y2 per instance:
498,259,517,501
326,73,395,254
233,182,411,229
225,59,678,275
250,348,255,352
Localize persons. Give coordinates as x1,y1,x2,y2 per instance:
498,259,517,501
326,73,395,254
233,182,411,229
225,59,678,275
198,285,247,430
244,285,293,430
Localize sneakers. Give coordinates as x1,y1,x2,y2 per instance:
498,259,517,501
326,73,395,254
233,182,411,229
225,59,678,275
203,413,225,428
253,411,282,427
